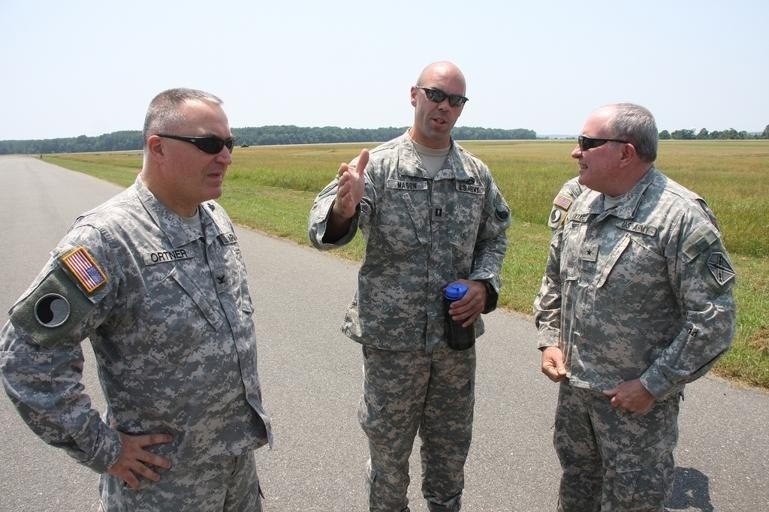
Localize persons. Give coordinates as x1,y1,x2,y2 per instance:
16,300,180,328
533,104,737,511
308,61,512,512
0,88,275,512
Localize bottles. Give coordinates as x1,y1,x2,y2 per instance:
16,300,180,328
444,284,476,351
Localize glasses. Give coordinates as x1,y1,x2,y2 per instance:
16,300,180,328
417,87,469,107
157,134,233,154
578,136,628,151
